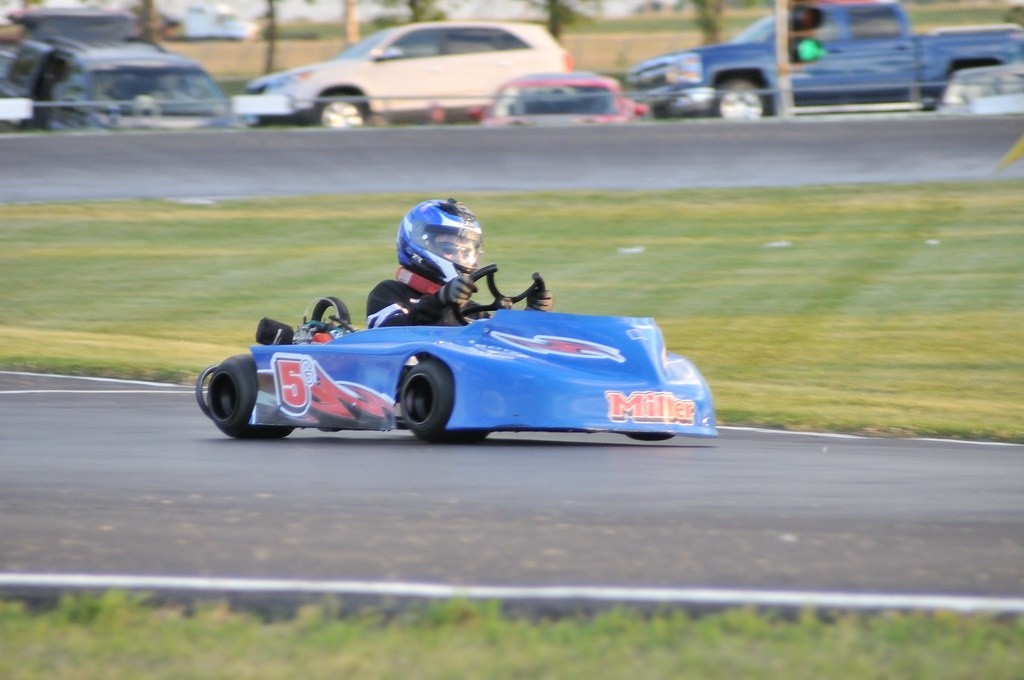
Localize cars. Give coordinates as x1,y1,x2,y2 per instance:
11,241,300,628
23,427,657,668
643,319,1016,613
469,72,649,126
233,21,573,129
0,5,236,128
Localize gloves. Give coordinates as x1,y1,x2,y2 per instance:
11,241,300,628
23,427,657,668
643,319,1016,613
421,276,478,318
526,289,553,311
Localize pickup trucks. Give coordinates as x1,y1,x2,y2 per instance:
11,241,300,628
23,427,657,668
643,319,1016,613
626,0,1024,121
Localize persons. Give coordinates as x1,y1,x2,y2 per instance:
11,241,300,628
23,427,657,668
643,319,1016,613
366,198,553,330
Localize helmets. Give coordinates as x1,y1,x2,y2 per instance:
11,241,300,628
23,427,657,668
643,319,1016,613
397,198,484,286
